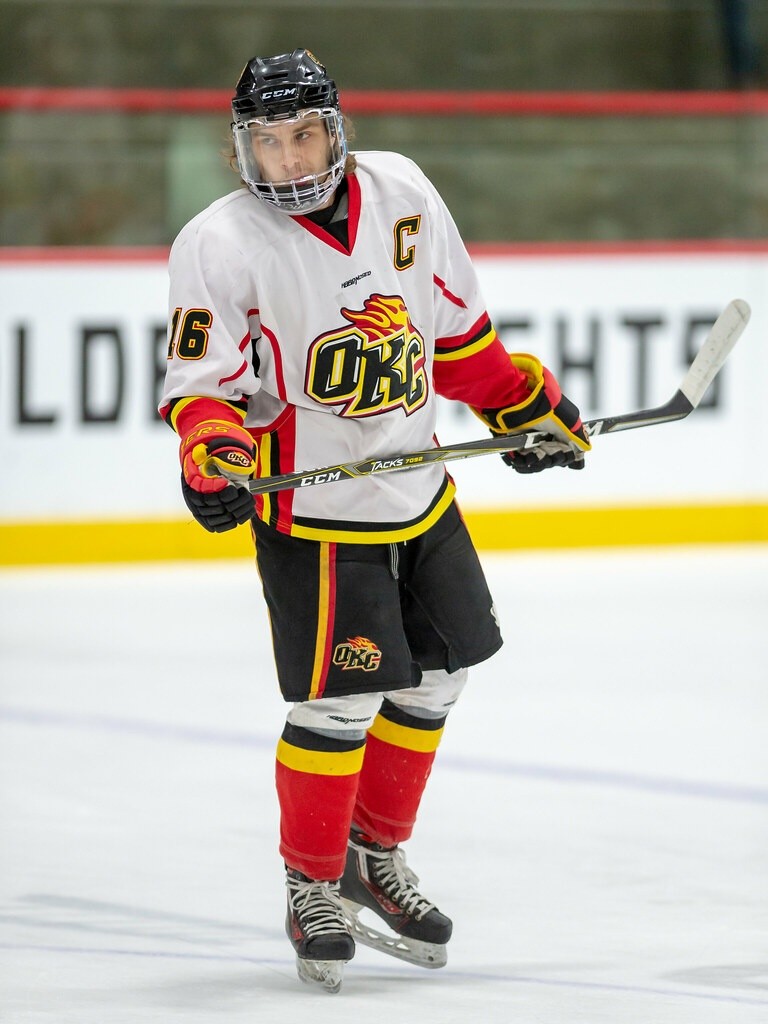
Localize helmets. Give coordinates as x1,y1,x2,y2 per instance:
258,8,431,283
229,47,341,211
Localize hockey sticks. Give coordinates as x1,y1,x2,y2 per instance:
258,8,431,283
232,299,750,497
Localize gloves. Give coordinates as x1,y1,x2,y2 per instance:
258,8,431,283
160,395,258,534
469,353,592,474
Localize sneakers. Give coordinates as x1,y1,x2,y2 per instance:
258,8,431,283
285,866,356,992
328,828,453,969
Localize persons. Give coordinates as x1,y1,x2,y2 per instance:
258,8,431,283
158,52,591,993
